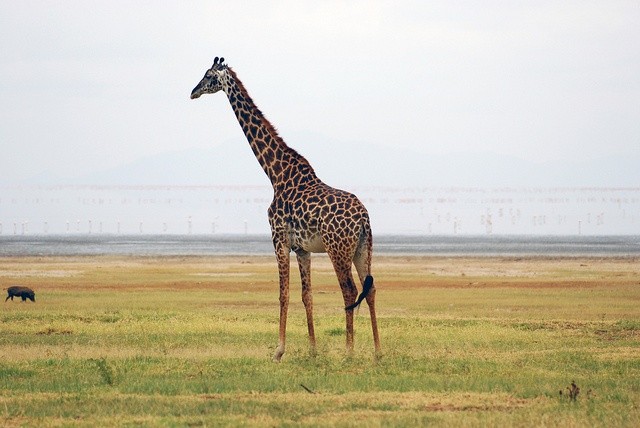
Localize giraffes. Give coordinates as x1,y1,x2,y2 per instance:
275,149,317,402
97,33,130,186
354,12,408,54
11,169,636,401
190,57,384,361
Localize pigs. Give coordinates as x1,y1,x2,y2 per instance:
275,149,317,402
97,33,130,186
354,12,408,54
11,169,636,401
4,284,35,303
